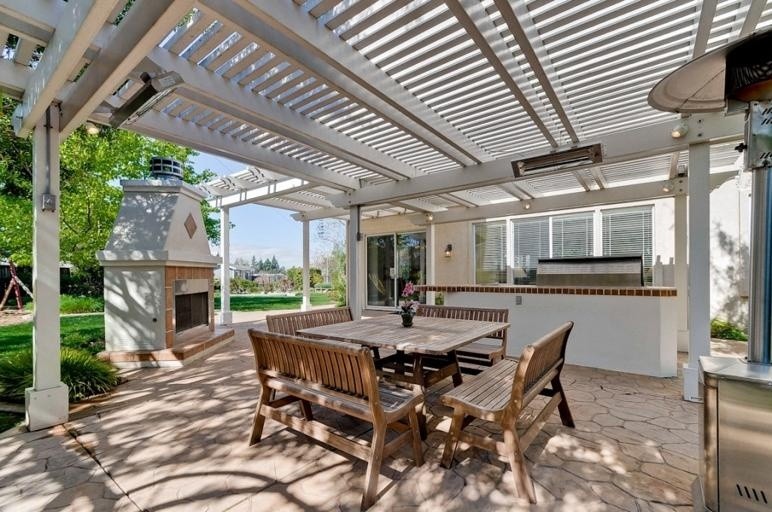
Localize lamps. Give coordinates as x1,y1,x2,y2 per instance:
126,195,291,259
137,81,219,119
670,123,689,139
520,199,531,210
663,183,673,192
444,244,452,258
423,210,435,224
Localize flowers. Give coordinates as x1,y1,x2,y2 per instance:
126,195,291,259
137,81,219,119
400,280,419,315
